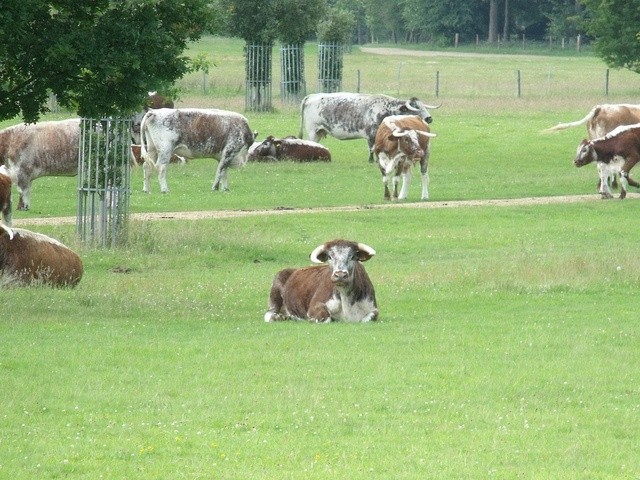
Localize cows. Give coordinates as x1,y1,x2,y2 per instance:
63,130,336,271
0,225,82,289
374,115,437,201
264,239,378,324
252,135,331,162
297,93,442,164
140,107,259,193
540,103,640,189
132,88,174,164
574,122,640,200
0,118,138,211
0,167,12,226
